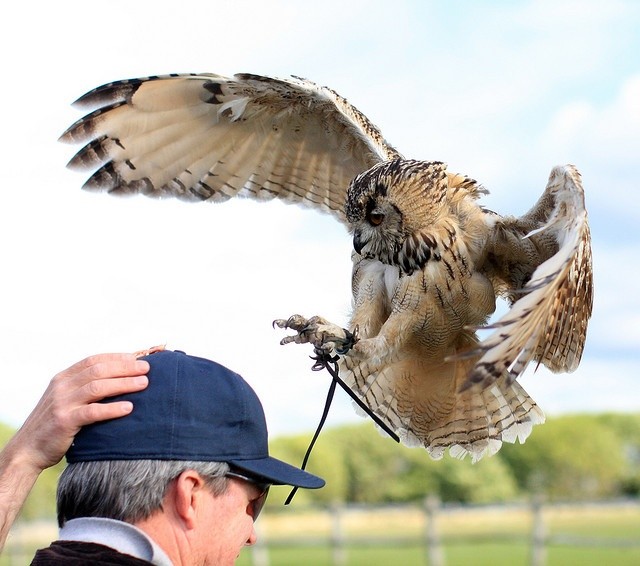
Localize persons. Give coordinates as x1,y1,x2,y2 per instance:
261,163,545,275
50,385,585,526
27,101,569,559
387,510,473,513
0,342,167,558
27,348,327,565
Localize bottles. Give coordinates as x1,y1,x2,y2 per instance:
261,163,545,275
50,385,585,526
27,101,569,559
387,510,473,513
65,350,326,489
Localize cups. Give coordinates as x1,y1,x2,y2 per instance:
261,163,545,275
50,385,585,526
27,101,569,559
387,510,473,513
225,471,269,523
